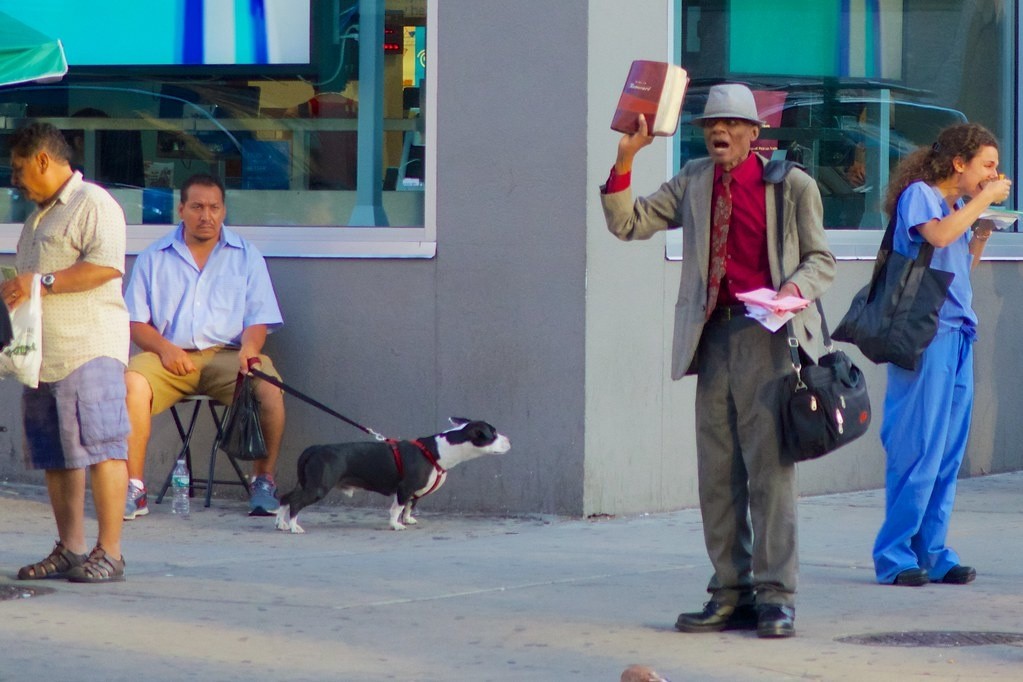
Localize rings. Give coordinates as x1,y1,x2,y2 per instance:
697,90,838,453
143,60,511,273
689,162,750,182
12,293,16,298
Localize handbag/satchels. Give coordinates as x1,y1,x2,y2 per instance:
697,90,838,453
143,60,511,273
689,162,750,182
782,351,871,460
0,273,42,388
219,375,268,460
831,181,955,371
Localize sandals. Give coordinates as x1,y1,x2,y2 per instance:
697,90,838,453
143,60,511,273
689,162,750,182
67,544,126,582
18,541,89,579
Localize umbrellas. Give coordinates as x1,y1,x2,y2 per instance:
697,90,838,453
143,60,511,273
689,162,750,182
0,10,69,86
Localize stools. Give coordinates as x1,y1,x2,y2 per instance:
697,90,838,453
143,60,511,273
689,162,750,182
155,394,252,508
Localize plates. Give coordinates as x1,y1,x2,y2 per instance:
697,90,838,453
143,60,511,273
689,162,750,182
981,208,1023,218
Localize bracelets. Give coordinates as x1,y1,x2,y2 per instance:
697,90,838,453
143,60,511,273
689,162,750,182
974,227,992,240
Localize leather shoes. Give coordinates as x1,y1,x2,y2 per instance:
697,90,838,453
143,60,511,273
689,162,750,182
943,564,975,583
757,602,795,636
675,602,756,631
895,568,929,585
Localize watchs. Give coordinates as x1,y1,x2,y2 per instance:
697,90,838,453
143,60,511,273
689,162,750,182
41,272,55,294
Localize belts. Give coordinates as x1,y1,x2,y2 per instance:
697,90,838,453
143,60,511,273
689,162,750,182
710,307,745,321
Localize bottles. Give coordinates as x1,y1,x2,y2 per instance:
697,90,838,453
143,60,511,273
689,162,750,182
172,460,190,515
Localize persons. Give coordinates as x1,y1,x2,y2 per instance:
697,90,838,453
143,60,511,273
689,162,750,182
0,122,131,583
282,52,362,191
872,121,1012,584
121,172,284,519
599,82,835,637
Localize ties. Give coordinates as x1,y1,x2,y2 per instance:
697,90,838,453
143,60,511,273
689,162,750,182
705,173,733,322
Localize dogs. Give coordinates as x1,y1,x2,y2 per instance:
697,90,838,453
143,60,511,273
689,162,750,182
275,415,512,532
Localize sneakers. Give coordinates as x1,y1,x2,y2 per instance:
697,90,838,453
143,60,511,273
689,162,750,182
248,473,280,515
124,481,149,519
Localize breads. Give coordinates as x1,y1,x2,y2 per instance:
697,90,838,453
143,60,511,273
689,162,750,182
979,174,1009,189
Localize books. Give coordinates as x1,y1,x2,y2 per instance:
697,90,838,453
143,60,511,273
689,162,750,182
611,60,690,136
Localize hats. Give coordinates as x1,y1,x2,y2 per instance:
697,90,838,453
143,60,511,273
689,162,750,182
688,84,766,127
